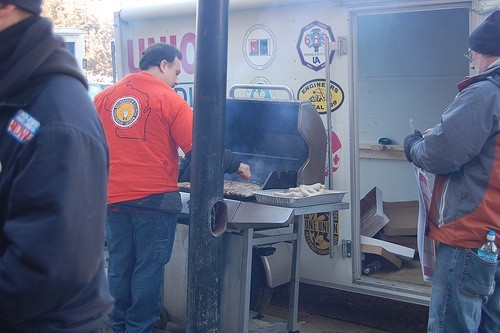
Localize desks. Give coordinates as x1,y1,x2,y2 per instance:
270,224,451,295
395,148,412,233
359,143,410,160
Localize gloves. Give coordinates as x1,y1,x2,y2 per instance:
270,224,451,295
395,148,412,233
401,128,423,163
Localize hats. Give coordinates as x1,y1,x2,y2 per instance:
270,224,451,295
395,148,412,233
467,9,500,55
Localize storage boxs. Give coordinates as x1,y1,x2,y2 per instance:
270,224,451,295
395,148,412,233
360,186,419,271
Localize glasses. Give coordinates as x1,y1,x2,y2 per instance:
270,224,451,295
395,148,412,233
464,49,473,63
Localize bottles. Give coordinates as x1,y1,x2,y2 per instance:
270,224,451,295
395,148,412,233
477,230,498,263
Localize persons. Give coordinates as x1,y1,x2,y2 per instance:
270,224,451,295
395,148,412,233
0,0,110,333
402,10,500,333
93,42,251,333
